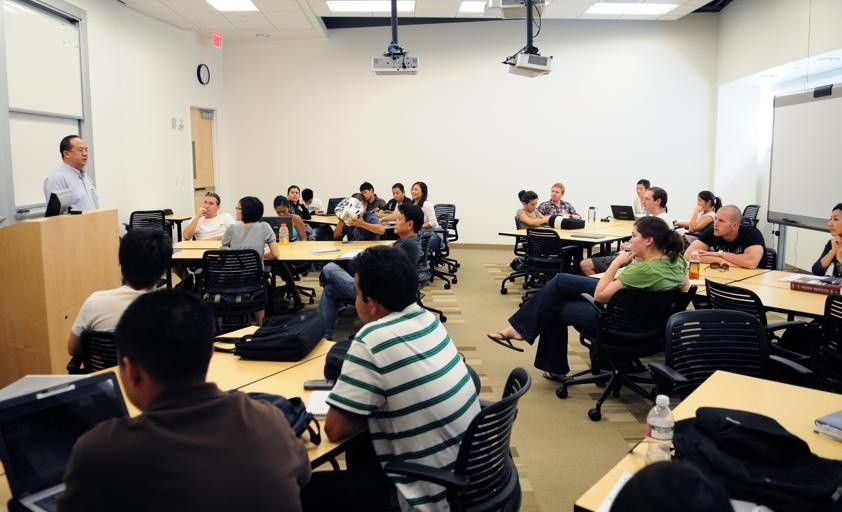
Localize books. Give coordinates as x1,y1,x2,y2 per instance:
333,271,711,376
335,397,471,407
790,276,842,296
815,411,841,437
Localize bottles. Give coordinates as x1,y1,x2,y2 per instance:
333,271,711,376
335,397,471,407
215,223,225,248
279,222,290,249
588,206,596,224
688,253,699,279
645,395,675,466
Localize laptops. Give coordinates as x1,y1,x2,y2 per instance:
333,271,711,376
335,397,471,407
611,205,637,220
260,216,296,243
0,371,132,512
316,197,345,216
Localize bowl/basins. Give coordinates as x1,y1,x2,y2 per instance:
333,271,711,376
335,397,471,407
334,197,366,228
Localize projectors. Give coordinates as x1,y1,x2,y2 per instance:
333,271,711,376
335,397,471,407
372,56,419,75
509,52,552,78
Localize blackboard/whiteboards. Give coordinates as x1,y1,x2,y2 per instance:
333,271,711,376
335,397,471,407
767,82,842,233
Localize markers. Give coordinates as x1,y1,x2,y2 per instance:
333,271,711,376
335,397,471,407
783,219,798,224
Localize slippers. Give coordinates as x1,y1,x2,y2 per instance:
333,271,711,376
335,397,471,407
543,371,574,382
487,333,524,352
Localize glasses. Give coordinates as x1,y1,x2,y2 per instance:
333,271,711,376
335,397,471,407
704,261,730,273
208,192,217,196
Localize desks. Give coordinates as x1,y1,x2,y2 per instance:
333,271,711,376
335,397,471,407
573,370,840,511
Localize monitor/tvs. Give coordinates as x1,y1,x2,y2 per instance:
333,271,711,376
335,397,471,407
45,189,73,216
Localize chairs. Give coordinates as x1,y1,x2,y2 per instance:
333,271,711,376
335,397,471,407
372,367,533,512
1,324,465,512
498,200,841,422
129,198,461,324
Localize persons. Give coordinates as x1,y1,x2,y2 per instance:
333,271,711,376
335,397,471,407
44,136,97,215
812,204,842,277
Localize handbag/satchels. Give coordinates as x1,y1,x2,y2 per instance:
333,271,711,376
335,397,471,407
550,214,585,229
672,405,841,512
234,311,326,362
248,392,321,446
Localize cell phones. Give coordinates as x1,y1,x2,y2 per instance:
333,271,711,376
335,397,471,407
303,380,334,390
